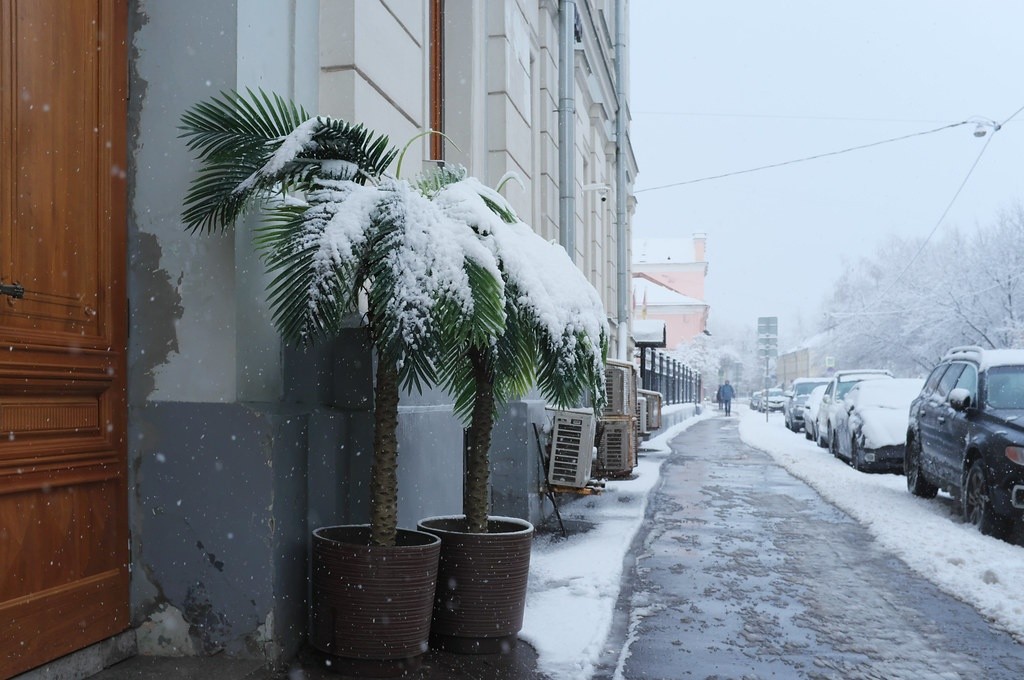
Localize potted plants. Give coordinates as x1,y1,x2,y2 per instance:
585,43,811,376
175,85,612,679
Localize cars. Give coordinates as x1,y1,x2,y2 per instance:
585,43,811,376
832,378,925,473
804,369,894,454
749,387,783,413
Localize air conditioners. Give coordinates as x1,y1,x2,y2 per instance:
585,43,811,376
548,359,663,488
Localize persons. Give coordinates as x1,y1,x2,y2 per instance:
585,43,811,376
717,383,724,410
720,380,736,416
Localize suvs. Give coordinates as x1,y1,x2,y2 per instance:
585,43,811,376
903,345,1024,542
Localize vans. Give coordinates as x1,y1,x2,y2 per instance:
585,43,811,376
782,378,832,432
